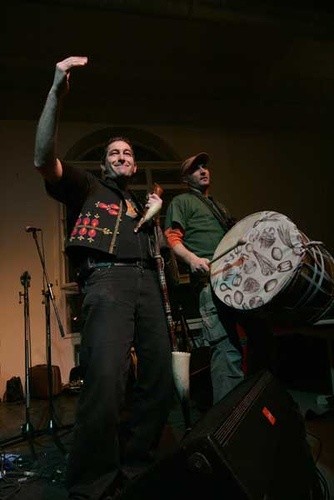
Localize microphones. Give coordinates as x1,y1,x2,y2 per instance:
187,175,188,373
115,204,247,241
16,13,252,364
25,226,42,232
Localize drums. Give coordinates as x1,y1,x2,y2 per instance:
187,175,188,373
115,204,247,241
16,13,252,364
210,210,334,329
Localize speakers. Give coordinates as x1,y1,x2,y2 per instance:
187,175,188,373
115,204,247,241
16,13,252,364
122,369,318,500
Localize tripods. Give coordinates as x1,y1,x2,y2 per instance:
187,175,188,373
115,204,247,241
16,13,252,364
0,232,75,458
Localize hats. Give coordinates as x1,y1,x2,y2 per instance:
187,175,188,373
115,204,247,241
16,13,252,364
180,152,209,179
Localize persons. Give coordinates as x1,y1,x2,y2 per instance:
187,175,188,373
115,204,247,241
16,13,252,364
165,151,271,408
32,56,185,500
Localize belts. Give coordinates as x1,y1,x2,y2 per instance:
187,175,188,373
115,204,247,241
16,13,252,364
84,259,154,269
